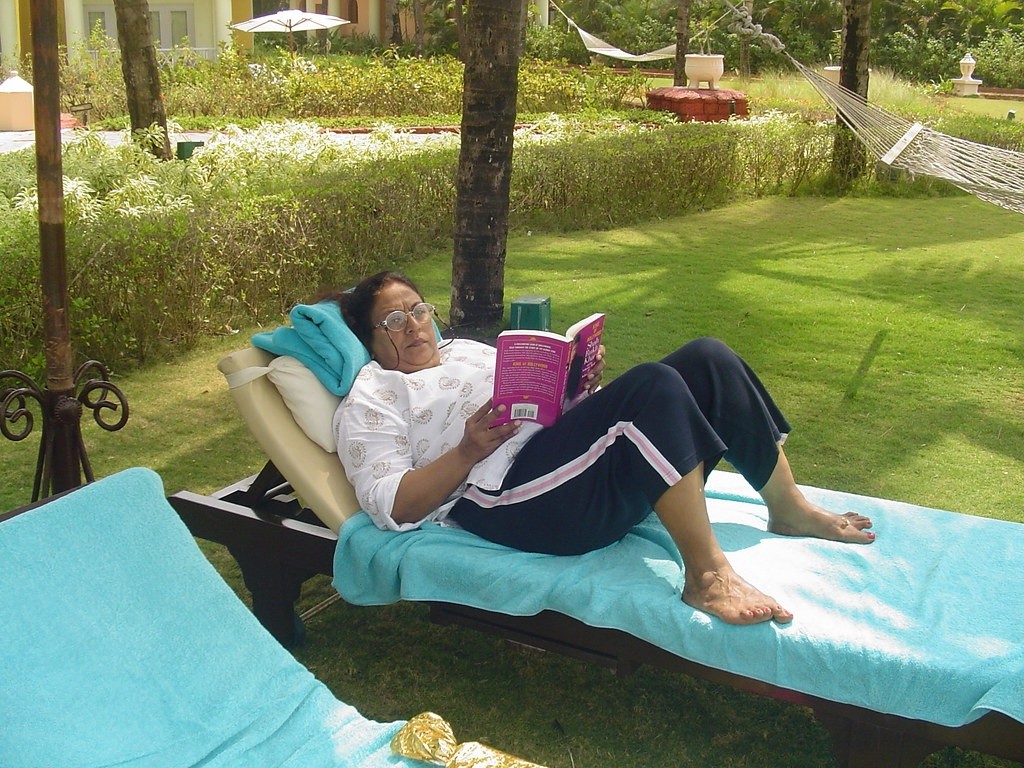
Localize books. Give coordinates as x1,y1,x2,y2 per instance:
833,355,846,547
491,313,606,427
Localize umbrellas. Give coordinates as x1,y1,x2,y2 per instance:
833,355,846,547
231,10,350,69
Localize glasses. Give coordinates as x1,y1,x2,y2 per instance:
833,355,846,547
372,302,435,332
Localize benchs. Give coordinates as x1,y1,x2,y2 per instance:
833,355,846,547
166,282,1023,768
1,466,548,768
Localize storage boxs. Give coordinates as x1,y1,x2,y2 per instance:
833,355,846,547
177,142,204,160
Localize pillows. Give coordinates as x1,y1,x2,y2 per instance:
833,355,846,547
267,354,341,454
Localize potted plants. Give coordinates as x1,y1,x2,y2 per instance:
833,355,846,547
824,30,872,83
684,20,725,90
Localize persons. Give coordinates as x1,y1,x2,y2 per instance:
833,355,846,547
337,271,877,624
562,336,584,415
